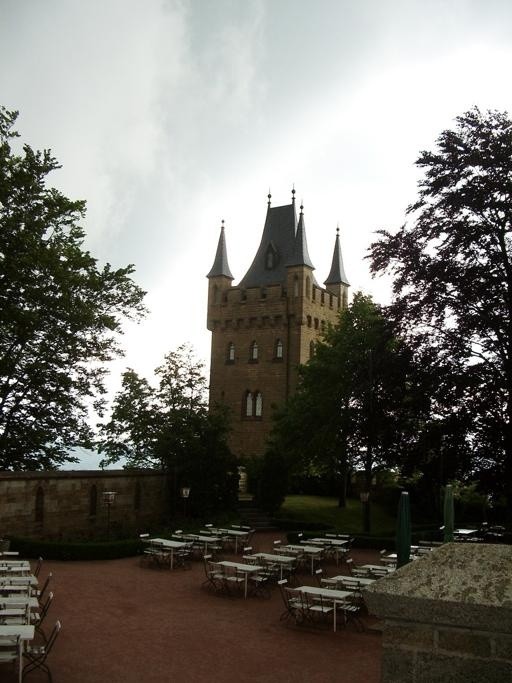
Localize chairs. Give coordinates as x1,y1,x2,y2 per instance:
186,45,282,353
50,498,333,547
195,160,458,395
139,521,256,571
19,556,61,683
408,522,506,561
277,548,399,631
202,530,355,601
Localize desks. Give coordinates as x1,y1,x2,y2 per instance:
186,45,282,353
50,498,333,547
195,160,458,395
0,552,40,682
